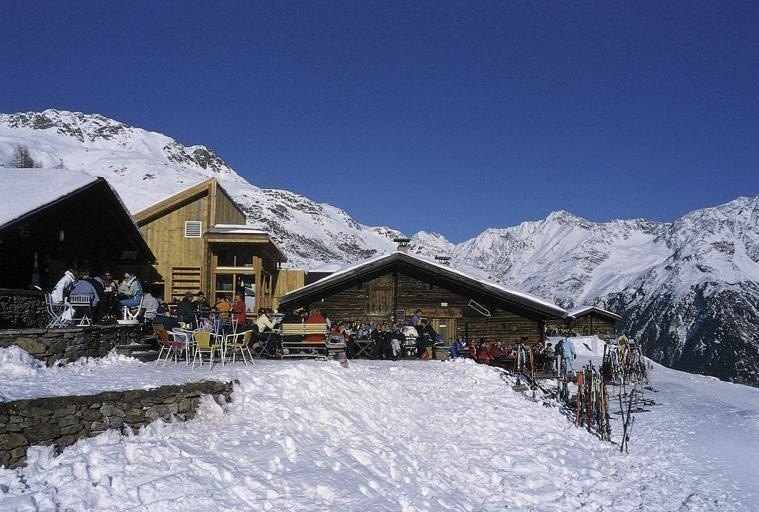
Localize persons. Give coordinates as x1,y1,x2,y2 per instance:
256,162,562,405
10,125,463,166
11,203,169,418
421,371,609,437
45,262,340,366
338,310,556,379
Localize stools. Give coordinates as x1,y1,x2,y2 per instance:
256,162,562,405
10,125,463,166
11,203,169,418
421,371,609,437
431,345,450,361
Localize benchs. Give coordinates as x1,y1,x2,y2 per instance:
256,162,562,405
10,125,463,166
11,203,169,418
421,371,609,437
281,323,327,359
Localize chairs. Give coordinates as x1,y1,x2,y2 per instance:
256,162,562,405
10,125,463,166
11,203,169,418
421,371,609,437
44,279,248,372
249,322,275,357
387,333,419,360
